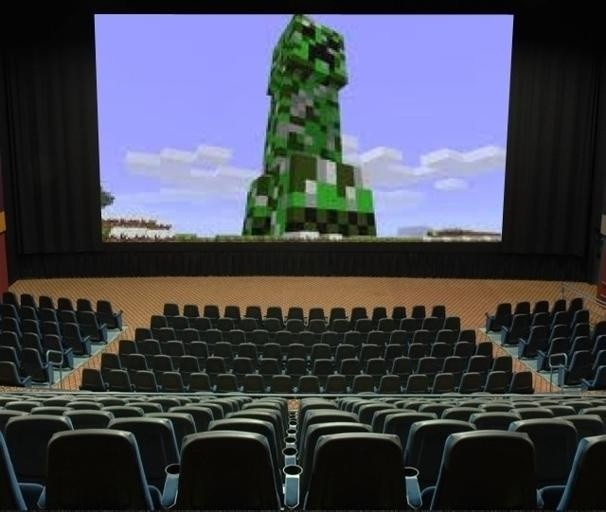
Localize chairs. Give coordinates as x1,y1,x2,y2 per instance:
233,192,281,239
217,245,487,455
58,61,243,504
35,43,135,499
0,293,123,387
485,298,606,391
